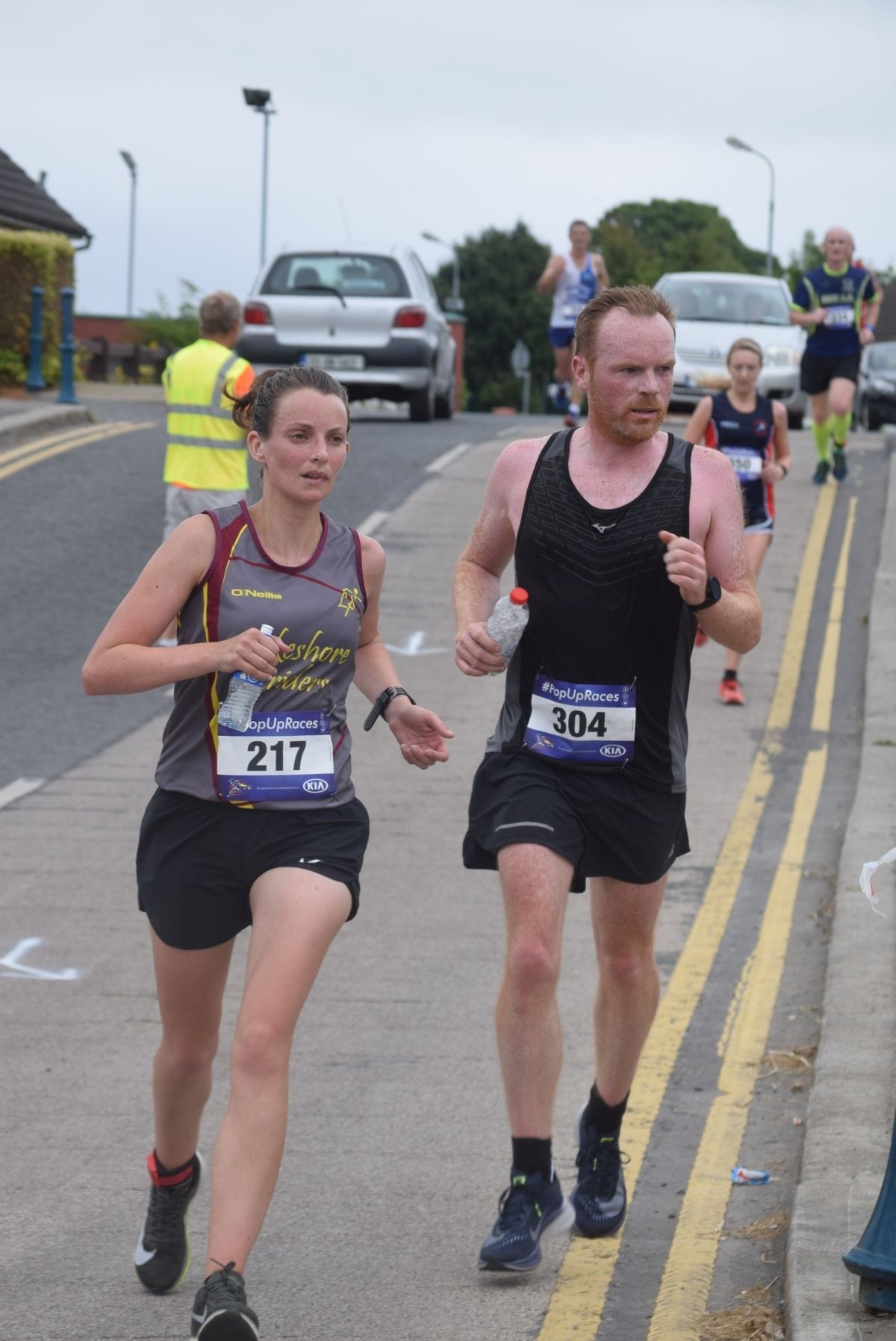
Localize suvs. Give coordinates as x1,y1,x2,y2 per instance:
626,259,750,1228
235,249,455,422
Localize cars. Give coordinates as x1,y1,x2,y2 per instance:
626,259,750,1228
853,341,896,432
650,270,810,432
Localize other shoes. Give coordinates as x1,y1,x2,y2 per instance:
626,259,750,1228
813,459,830,483
557,387,567,407
566,411,580,427
833,449,848,481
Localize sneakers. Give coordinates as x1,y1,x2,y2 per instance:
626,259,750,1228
719,678,744,705
134,1150,205,1293
573,1104,632,1236
479,1164,563,1271
695,623,708,648
190,1258,260,1341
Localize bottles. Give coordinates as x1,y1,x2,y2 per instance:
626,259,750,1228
479,588,531,676
216,624,274,732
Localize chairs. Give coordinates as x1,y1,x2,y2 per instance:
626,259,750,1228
293,267,318,291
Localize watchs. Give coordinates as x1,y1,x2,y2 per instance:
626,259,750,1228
686,574,722,612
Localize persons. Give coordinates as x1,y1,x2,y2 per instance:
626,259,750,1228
453,283,760,1272
537,221,882,706
148,292,257,639
77,363,455,1341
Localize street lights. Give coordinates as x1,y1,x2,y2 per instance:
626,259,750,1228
239,86,275,278
725,134,777,273
422,232,460,298
118,150,139,316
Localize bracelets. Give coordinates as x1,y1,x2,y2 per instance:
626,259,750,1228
362,686,417,731
864,324,874,331
